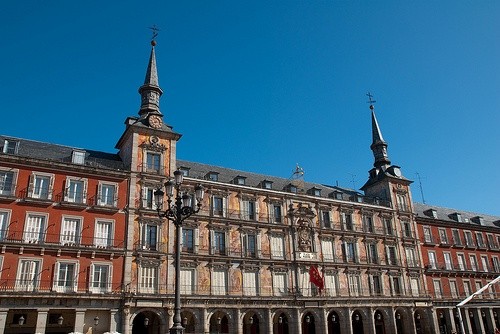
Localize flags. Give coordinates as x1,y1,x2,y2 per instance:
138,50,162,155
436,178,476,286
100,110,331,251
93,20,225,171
309,264,324,290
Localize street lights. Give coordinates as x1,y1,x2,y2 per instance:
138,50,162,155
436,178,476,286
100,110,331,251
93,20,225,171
153,166,206,333
